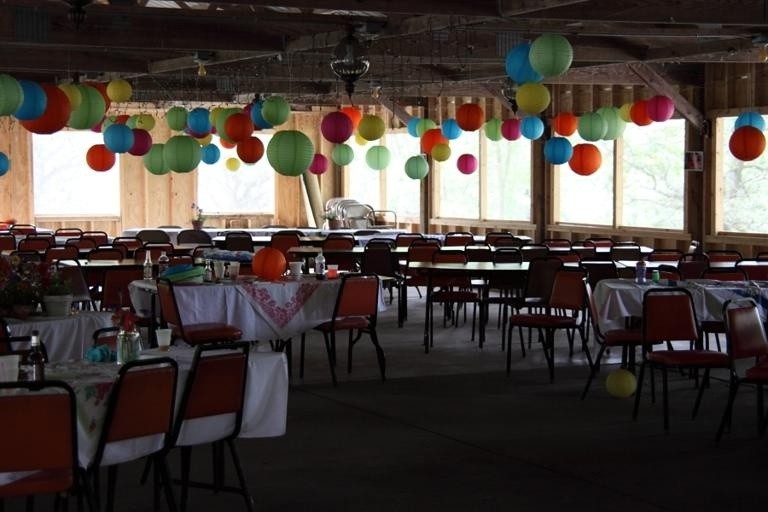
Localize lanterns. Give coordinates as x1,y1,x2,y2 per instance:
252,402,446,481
267,107,391,176
729,112,765,161
0,74,155,171
605,369,637,399
405,33,675,179
143,95,290,175
252,248,286,281
0,151,8,176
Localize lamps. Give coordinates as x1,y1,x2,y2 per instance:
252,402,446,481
193,51,211,77
330,24,370,96
501,77,523,115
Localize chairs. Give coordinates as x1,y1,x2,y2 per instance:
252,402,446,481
0,198,768,512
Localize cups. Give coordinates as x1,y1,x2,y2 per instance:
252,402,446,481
213,262,225,278
326,264,338,278
226,262,241,281
288,261,302,276
154,328,172,352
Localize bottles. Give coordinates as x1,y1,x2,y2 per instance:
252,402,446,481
204,258,212,282
26,329,44,392
635,258,646,283
143,250,153,280
158,250,169,275
650,269,659,281
313,250,326,275
